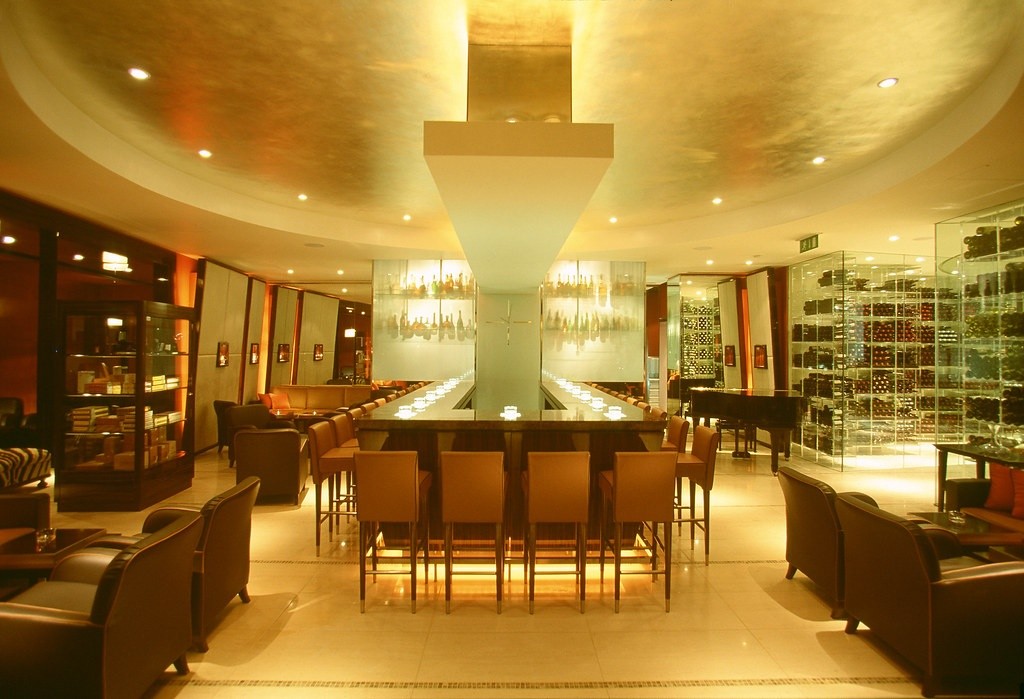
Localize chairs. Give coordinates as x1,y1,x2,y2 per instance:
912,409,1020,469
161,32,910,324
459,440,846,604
311,374,721,616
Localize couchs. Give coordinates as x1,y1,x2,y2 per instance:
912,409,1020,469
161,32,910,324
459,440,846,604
778,466,1024,699
0,383,371,699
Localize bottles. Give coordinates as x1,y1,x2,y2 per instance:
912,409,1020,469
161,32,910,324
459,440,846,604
791,214,1023,454
541,274,643,297
377,312,474,340
680,299,722,381
543,310,639,343
377,273,474,297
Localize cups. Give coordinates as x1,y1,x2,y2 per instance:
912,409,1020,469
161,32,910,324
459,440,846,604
396,379,456,415
504,406,517,414
556,379,621,414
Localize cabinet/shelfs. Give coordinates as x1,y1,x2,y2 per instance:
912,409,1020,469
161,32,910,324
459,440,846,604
68,294,195,474
679,295,723,378
785,252,954,460
933,198,1023,439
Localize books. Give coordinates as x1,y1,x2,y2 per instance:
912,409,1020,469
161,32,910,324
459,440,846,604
70,406,182,433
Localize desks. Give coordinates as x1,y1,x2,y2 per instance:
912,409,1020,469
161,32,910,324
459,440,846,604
267,406,331,432
933,440,1024,533
1,522,104,588
358,367,663,553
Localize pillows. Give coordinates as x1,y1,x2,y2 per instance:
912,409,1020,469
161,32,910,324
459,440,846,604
984,465,1015,508
258,392,272,410
270,393,292,410
1011,470,1024,518
